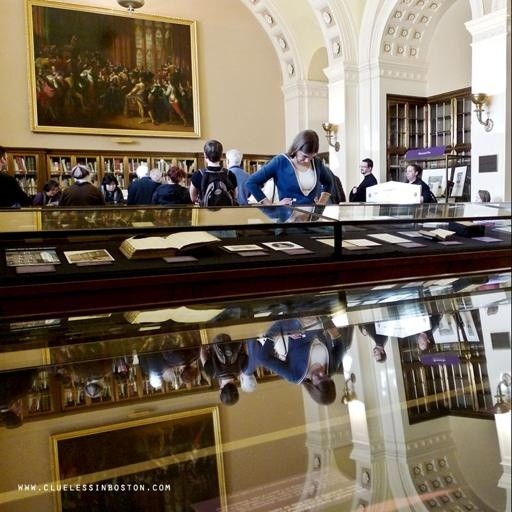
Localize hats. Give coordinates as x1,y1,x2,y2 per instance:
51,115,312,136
71,165,89,179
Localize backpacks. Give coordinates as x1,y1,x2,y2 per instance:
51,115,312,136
198,166,234,206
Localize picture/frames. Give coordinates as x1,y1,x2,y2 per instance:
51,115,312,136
24,1,201,140
49,404,229,512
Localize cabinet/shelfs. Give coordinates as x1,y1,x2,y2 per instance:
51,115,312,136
0,146,293,206
0,336,342,426
397,308,496,426
386,86,472,184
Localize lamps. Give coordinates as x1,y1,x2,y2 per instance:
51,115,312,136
322,123,340,152
116,0,145,12
489,373,511,414
471,93,493,132
341,373,356,404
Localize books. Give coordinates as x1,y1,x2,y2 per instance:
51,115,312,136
418,228,456,241
126,307,226,327
120,230,220,260
422,277,460,288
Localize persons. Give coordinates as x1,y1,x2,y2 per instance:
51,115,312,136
0,336,268,430
319,164,340,200
58,410,218,511
351,156,379,203
404,165,436,205
35,39,193,127
361,321,390,363
323,329,353,357
246,131,332,204
250,322,346,403
416,314,443,350
1,140,279,211
